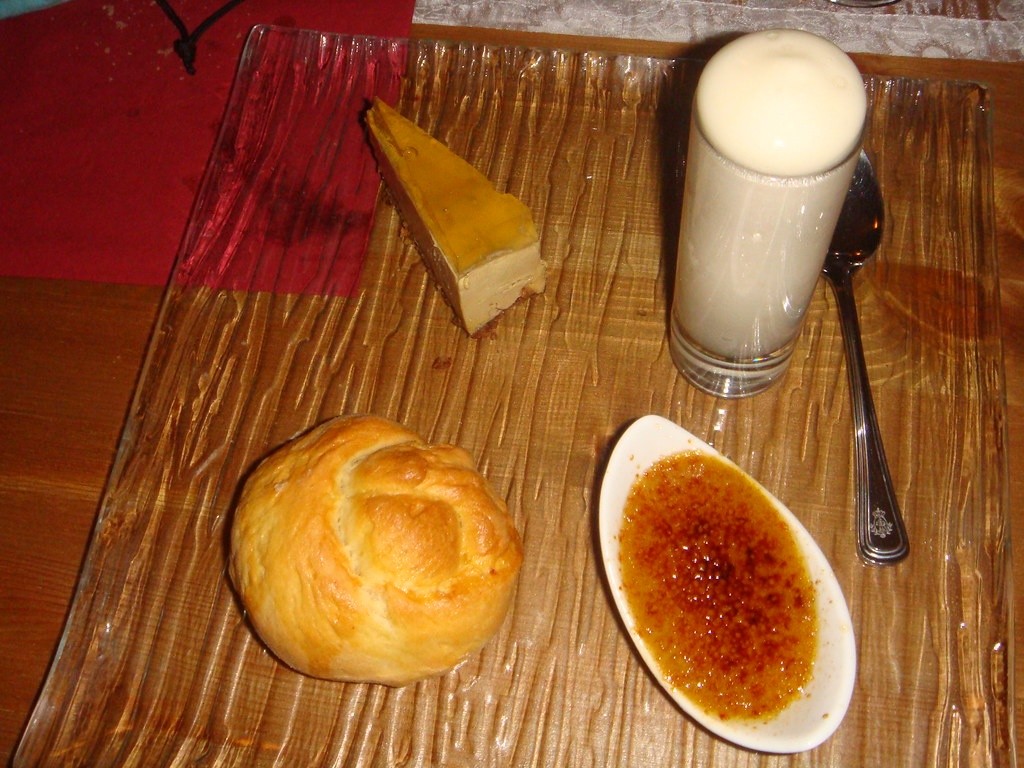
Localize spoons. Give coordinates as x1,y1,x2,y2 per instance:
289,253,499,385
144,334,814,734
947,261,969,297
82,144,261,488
820,148,910,566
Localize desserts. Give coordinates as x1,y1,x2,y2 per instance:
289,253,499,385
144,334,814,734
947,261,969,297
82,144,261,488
364,95,548,335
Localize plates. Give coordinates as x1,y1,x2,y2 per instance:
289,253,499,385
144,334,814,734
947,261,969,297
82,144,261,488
599,413,857,755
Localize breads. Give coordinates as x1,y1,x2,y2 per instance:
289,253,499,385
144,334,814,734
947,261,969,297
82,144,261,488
227,413,523,686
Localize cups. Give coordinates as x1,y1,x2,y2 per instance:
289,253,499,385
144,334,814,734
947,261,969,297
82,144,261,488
669,28,867,399
